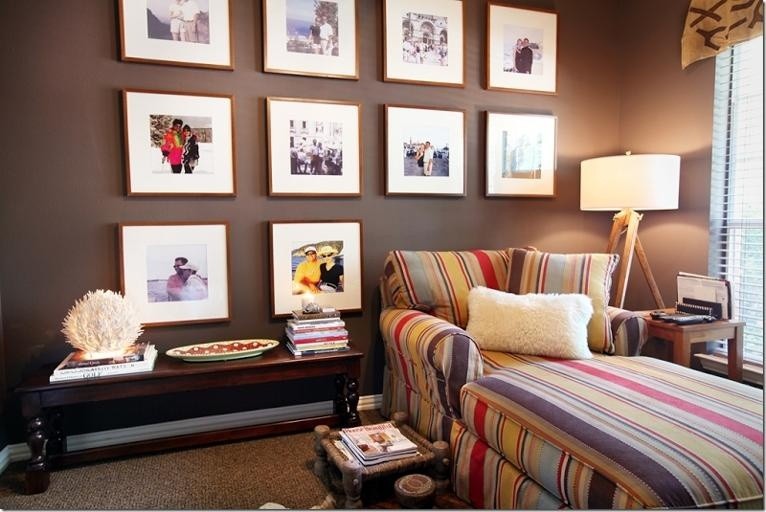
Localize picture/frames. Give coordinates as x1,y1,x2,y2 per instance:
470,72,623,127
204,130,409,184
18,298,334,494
266,96,364,198
262,0,360,80
486,0,560,97
270,219,366,319
121,88,239,197
118,0,235,71
385,103,468,197
117,221,233,328
485,110,557,198
383,0,467,89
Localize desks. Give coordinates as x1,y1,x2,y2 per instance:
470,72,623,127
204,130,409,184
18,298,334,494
14,337,365,495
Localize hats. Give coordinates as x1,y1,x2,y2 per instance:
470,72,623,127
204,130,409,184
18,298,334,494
317,246,338,258
179,261,200,271
304,246,317,254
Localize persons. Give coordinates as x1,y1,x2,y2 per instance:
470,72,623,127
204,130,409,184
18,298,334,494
512,37,533,74
308,16,334,56
291,136,342,174
414,140,434,175
160,119,199,174
166,256,207,300
293,245,344,294
168,0,199,42
403,36,447,64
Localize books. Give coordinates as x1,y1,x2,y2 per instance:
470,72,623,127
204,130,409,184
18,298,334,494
338,421,423,468
284,307,352,356
48,340,159,384
676,272,733,322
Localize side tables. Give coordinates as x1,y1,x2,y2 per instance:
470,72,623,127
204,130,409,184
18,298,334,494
641,309,745,384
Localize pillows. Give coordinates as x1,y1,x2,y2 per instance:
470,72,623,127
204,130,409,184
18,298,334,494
465,286,594,361
507,248,620,355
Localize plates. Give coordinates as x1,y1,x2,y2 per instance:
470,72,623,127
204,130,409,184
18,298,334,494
165,339,280,363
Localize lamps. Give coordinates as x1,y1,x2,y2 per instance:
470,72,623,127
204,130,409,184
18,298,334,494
58,289,145,359
581,150,681,309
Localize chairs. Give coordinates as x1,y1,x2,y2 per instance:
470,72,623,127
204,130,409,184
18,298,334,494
379,248,649,441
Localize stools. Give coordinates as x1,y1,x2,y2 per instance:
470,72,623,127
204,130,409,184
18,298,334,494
459,356,764,511
313,410,451,510
